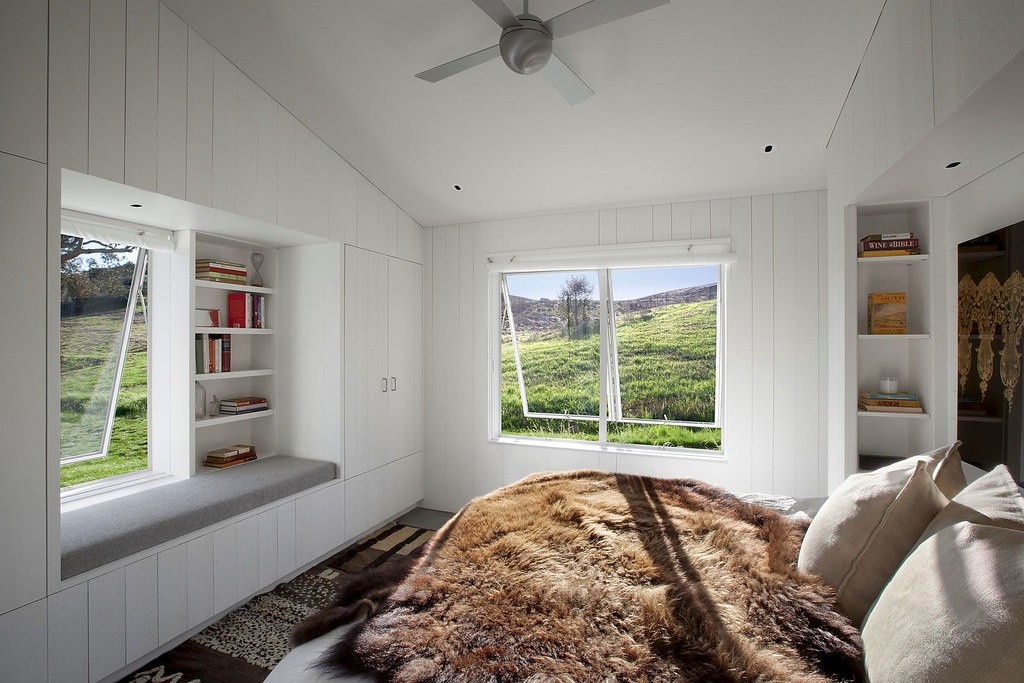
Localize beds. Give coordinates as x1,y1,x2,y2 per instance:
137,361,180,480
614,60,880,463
262,469,868,683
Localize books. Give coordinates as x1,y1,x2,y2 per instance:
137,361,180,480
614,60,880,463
195,258,249,286
203,444,258,469
858,389,923,414
219,397,268,415
858,232,919,258
867,291,908,335
195,333,231,374
227,292,267,329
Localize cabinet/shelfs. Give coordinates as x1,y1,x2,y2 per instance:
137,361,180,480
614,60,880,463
276,244,420,479
856,254,932,419
171,229,277,475
958,249,1007,422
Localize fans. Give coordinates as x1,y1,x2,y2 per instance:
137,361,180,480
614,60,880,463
416,0,670,106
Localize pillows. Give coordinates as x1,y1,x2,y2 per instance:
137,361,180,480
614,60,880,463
858,464,1024,683
796,440,967,631
